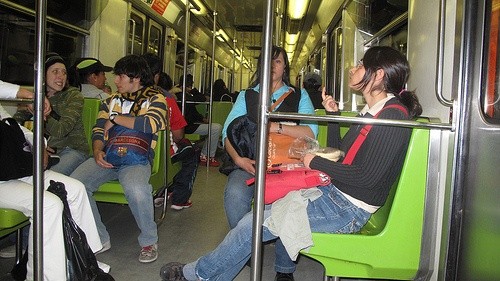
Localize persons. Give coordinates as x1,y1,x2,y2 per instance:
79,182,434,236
69,58,114,100
142,53,199,210
301,72,339,109
174,73,223,167
221,45,319,281
159,45,423,281
70,55,170,262
12,52,90,177
0,79,112,281
209,79,236,104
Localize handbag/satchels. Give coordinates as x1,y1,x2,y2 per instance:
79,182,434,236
0,121,61,181
12,104,52,142
267,131,339,170
264,170,332,206
102,117,150,164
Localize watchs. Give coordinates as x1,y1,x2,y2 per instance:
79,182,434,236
109,115,118,126
277,124,283,134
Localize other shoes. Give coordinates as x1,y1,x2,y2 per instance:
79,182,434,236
246,257,251,268
160,262,189,281
170,201,193,210
137,241,158,263
94,240,112,256
200,153,210,165
275,270,296,281
209,157,221,167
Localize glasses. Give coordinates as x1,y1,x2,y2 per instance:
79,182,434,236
357,59,363,67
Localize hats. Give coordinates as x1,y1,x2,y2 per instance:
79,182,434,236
44,50,67,121
303,74,323,85
72,57,113,72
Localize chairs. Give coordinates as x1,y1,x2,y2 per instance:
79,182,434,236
0,100,442,281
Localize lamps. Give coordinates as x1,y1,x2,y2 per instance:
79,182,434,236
210,29,230,43
228,45,251,69
283,0,312,65
180,0,208,17
